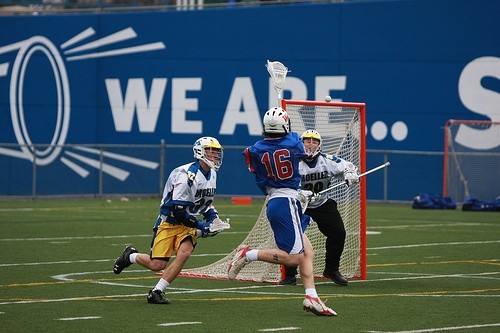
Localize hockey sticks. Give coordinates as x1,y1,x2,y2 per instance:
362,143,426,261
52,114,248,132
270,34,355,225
265,59,292,108
298,161,391,215
208,218,230,232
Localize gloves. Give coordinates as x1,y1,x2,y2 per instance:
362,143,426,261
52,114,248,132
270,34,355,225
195,219,213,233
300,190,321,204
205,211,219,223
343,171,359,188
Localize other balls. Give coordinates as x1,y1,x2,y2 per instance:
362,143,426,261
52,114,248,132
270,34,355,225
325,95,331,102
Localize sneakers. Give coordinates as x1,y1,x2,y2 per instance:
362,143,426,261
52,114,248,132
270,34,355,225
146,289,171,304
228,243,253,281
302,294,338,316
112,245,138,274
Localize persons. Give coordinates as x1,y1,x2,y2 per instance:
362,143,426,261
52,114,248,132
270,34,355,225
228,106,336,316
114,136,230,304
279,128,358,284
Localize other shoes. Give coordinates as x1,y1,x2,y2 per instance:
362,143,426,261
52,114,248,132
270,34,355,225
279,274,297,286
323,269,349,285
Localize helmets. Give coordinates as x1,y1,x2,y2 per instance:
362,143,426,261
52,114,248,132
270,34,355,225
300,129,322,164
263,107,291,134
193,137,223,172
